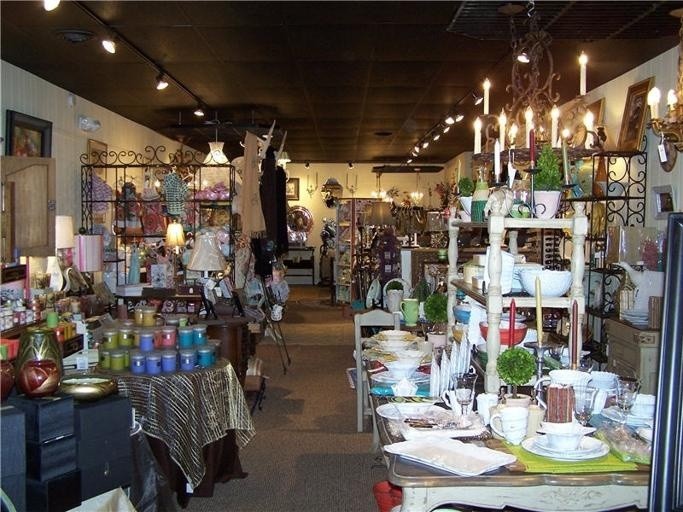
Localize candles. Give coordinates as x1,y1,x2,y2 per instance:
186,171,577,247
584,123,593,149
534,276,543,342
650,104,659,119
572,300,578,369
474,128,481,154
509,133,517,149
483,88,489,114
499,125,505,152
529,129,534,167
494,142,500,175
561,138,571,185
669,104,675,110
508,299,516,347
551,119,558,148
579,62,586,95
525,120,531,148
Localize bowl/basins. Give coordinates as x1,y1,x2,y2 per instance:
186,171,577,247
538,427,596,450
477,321,528,347
519,269,573,298
590,370,618,388
379,330,410,340
392,402,432,417
384,361,419,380
629,394,655,417
372,336,418,350
390,385,418,396
452,304,471,324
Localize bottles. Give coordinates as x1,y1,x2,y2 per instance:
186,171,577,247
0,312,5,331
208,345,216,363
134,306,144,325
180,351,195,371
17,297,26,307
193,324,208,345
166,317,179,327
196,345,211,367
111,352,125,371
161,354,178,373
125,350,130,367
152,329,163,349
133,329,141,346
101,352,111,369
139,332,155,352
188,302,195,313
26,308,34,323
4,312,16,329
178,313,189,327
15,306,26,327
102,331,118,349
129,355,146,375
144,309,156,326
163,326,176,347
118,327,135,348
178,325,194,349
146,354,162,375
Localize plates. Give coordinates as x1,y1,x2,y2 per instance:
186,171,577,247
500,312,527,323
370,370,432,384
521,435,609,461
534,438,604,454
383,437,517,478
366,279,381,309
397,420,487,440
376,401,447,420
537,426,596,436
370,387,430,399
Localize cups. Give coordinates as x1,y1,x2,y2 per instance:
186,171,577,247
490,407,528,447
452,372,478,418
443,390,475,417
400,299,418,326
615,379,637,418
476,393,500,424
573,385,599,427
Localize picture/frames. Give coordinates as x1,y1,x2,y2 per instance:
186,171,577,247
585,97,606,149
286,178,299,201
5,109,53,158
615,75,656,152
87,138,107,182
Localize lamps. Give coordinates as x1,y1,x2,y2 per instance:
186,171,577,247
348,163,354,169
278,152,291,164
73,234,104,276
431,132,440,141
424,212,448,236
203,127,230,165
406,158,412,163
155,77,169,90
412,151,418,157
472,93,483,105
445,116,454,124
367,201,394,230
440,124,449,133
415,146,421,153
455,112,464,122
304,162,310,169
103,40,117,54
194,109,204,116
421,141,429,149
55,215,76,257
187,237,227,298
42,0,60,11
164,222,186,282
518,52,529,63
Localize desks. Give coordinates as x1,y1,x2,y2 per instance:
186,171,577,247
112,282,202,301
56,356,256,509
208,316,247,378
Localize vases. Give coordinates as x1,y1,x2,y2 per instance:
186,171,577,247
0,360,16,400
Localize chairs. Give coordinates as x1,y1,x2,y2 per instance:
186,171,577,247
355,311,400,433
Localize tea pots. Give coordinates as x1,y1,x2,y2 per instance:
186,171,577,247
534,369,592,412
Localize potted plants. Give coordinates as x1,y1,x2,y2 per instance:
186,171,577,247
18,337,61,397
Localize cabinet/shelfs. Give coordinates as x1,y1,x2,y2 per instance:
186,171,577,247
446,202,588,394
335,198,382,319
584,150,648,372
82,165,236,297
282,247,320,286
